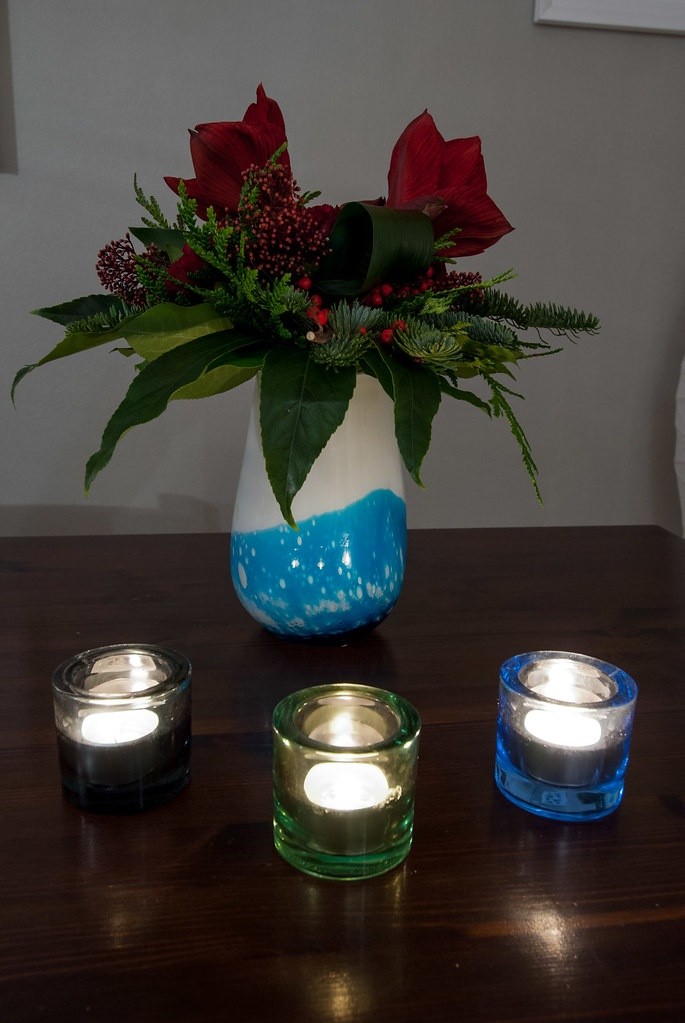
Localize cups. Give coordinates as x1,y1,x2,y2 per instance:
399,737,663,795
54,643,192,813
272,682,420,880
495,651,638,821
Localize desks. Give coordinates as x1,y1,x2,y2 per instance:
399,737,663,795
0,518,685,1023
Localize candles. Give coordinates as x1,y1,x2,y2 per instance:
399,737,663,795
264,678,429,886
49,638,200,819
488,635,639,826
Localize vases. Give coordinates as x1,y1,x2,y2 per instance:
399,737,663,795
219,364,410,645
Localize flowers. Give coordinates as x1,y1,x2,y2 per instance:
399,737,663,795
9,77,609,531
